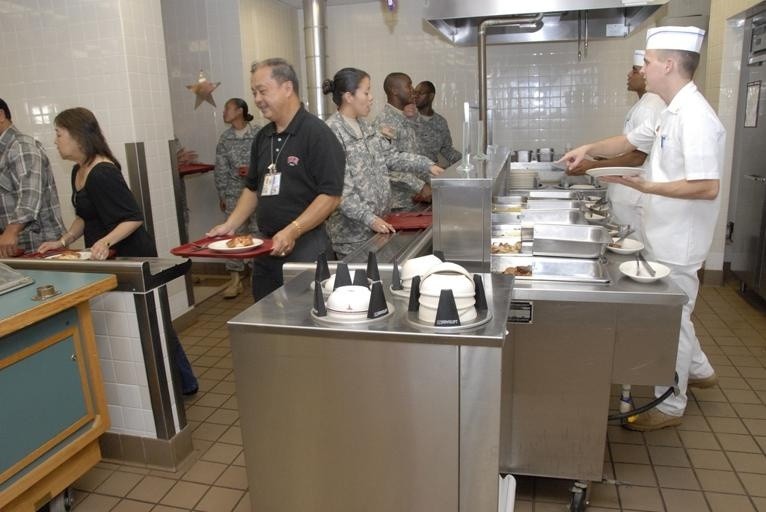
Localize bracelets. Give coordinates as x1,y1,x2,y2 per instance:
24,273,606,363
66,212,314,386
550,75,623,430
68,232,76,240
59,238,68,248
291,222,301,238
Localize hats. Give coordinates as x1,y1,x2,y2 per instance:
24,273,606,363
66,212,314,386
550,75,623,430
646,26,705,54
633,50,645,67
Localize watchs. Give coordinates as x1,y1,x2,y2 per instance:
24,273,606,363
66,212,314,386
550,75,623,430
98,239,111,248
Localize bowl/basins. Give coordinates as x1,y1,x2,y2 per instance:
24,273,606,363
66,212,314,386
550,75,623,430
618,260,671,286
418,272,479,324
582,203,610,223
400,254,444,295
322,284,373,314
323,270,369,292
606,236,646,255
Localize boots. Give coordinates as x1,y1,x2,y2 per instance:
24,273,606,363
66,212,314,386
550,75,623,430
222,271,244,298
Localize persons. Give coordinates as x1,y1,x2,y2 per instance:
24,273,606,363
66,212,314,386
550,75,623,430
215,98,265,297
403,81,462,170
205,57,346,303
36,107,198,396
372,73,432,209
323,68,444,261
566,50,668,245
553,25,727,432
0,98,68,259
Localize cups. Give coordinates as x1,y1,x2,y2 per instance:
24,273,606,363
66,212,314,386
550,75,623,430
564,144,572,154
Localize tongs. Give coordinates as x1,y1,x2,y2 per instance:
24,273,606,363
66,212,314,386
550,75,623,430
633,250,658,280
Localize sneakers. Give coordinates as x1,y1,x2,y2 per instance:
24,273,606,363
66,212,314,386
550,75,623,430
688,372,720,390
626,406,682,432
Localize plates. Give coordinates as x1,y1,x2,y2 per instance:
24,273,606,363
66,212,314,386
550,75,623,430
42,252,92,261
207,238,264,254
584,166,644,178
571,184,596,194
